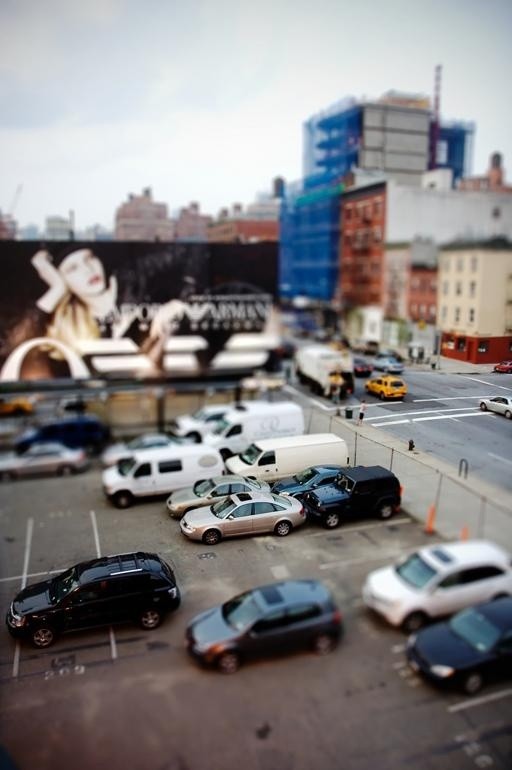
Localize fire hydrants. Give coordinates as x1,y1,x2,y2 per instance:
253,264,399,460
405,437,418,453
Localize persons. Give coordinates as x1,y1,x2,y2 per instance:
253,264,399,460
329,367,345,405
0,243,152,383
356,399,367,427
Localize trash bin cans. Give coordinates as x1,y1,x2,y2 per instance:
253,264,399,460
432,364,435,368
346,410,352,418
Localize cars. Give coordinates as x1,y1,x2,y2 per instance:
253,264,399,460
393,594,512,696
7,552,181,643
479,394,512,418
186,578,342,671
293,339,413,401
97,433,228,507
491,357,512,375
1,411,113,480
362,541,510,632
164,462,402,546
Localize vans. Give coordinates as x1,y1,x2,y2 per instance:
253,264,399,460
171,399,306,456
225,432,353,479
